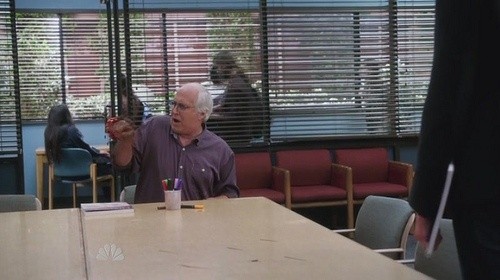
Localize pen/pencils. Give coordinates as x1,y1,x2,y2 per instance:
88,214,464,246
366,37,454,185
162,178,183,191
182,204,204,209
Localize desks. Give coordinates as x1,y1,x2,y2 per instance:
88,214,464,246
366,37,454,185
36,145,110,203
80,195,442,280
0,207,88,279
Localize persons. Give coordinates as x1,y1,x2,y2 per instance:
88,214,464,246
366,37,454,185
121,74,143,125
204,50,263,147
408,0,500,280
361,58,384,133
44,104,119,202
110,82,239,204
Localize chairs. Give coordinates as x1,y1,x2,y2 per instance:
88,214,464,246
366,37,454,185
234,147,465,280
48,147,115,208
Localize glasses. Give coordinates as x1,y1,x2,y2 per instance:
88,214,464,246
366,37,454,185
169,100,195,113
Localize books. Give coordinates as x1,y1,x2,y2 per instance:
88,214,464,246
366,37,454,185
81,202,135,216
424,163,453,258
